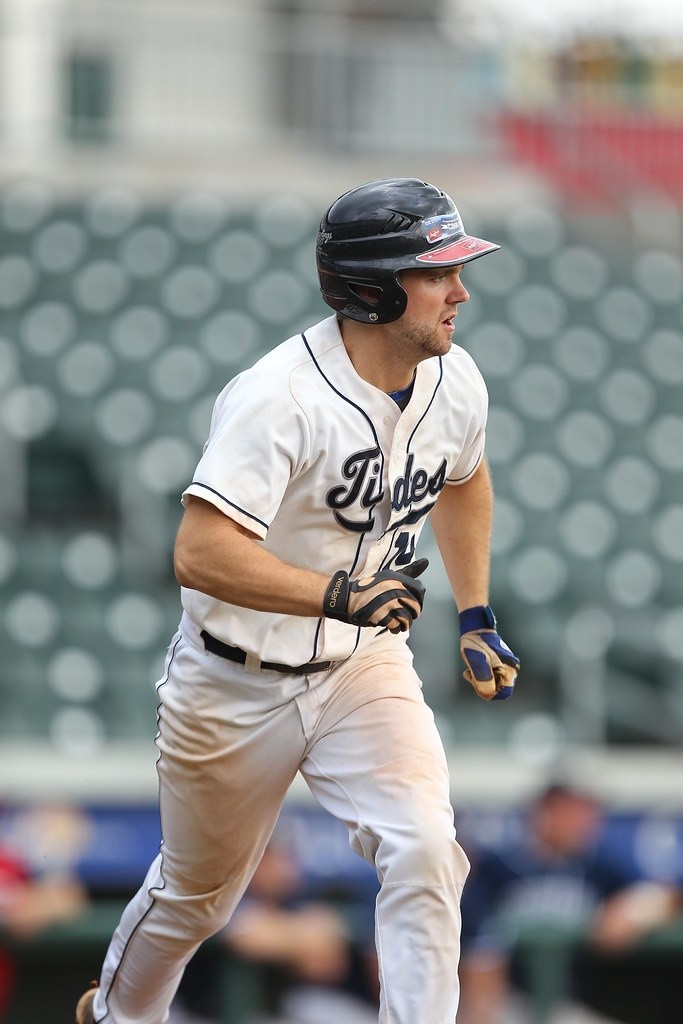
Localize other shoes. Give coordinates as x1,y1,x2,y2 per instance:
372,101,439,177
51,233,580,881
75,987,100,1024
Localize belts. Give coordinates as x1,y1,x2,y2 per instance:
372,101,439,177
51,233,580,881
200,630,336,675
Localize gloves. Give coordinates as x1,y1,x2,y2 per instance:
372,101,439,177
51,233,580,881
458,605,521,701
322,558,429,634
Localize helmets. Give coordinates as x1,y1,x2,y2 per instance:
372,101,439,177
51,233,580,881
316,177,502,324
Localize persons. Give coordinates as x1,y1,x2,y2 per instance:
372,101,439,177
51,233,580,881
0,804,91,1024
223,784,674,1024
78,179,517,1024
